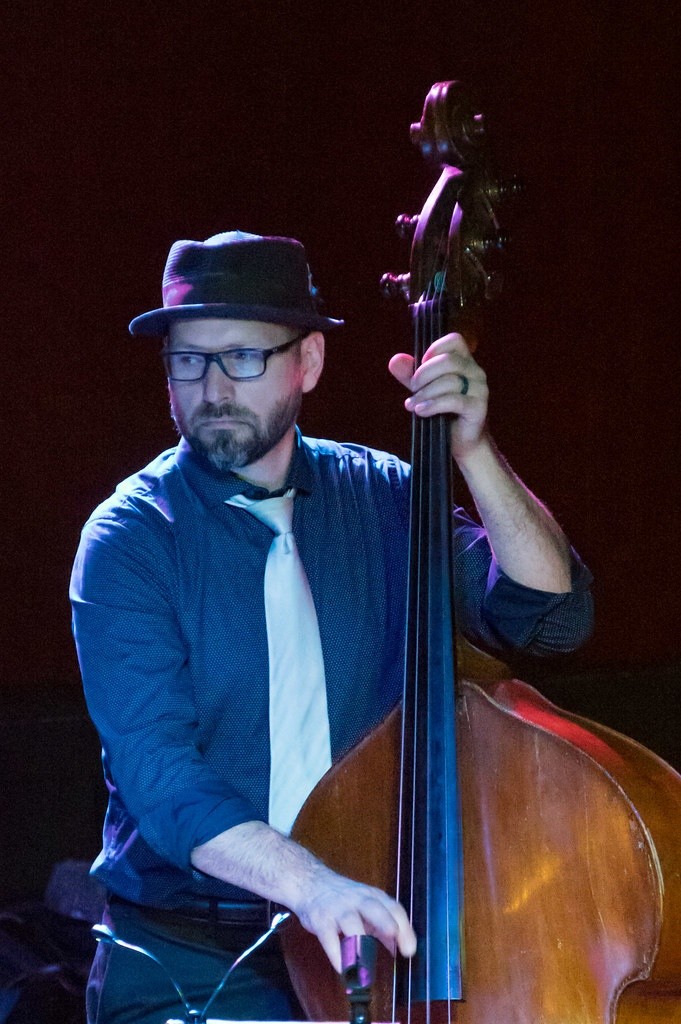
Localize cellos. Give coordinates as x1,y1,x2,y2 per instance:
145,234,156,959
271,78,679,1024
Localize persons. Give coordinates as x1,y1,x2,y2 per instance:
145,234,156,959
69,231,601,1024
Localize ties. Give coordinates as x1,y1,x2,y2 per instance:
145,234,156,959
226,492,329,836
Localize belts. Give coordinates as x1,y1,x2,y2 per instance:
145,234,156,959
104,896,279,956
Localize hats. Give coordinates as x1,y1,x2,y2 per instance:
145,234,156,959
129,231,346,331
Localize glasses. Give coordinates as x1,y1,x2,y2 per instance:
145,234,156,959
156,333,312,379
